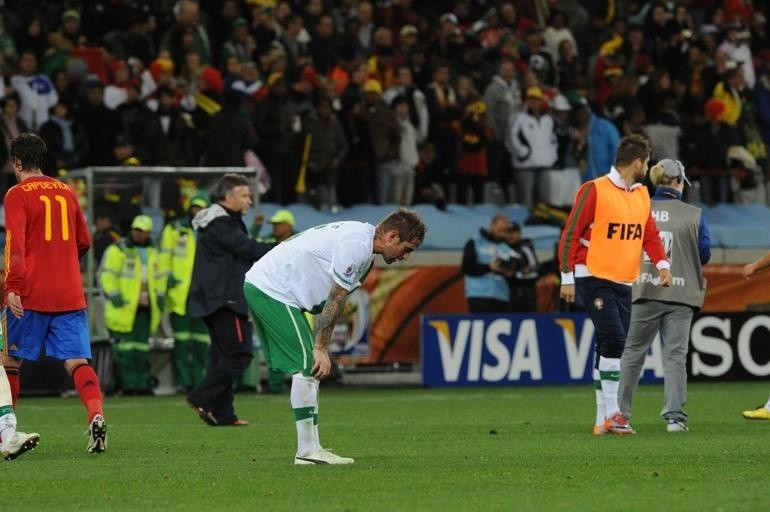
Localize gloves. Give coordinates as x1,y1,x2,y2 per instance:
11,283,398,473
108,292,131,310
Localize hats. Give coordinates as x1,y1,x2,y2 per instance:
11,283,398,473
130,213,155,233
268,208,298,227
52,9,751,124
190,190,211,208
508,222,521,232
649,158,693,189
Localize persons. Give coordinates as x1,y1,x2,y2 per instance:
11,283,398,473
460,213,540,320
241,207,430,467
556,134,674,436
0,131,109,454
617,158,713,432
0,0,770,212
740,249,769,420
185,172,279,425
1,317,41,460
85,190,296,398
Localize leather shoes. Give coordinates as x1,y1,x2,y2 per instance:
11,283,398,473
183,396,251,427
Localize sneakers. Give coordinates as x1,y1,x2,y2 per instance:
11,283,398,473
592,412,637,437
740,405,770,421
293,447,357,468
84,413,110,456
0,429,42,463
664,418,691,433
